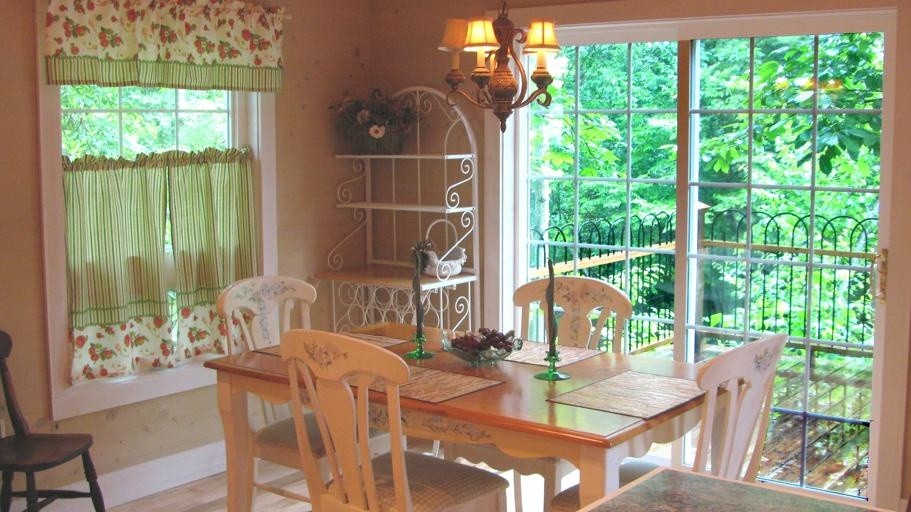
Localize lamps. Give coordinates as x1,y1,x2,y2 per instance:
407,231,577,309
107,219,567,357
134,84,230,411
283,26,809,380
436,0,565,133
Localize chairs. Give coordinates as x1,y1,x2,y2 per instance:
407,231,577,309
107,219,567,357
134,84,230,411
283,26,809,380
0,324,107,512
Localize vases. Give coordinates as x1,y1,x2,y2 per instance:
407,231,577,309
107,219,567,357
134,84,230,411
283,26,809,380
353,124,403,155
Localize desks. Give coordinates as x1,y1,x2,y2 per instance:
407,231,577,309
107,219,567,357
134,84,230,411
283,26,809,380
201,320,750,512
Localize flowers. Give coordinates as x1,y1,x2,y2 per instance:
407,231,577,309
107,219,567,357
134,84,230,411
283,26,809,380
320,86,432,148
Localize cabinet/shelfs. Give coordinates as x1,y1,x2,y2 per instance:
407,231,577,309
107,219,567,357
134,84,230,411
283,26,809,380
310,81,477,335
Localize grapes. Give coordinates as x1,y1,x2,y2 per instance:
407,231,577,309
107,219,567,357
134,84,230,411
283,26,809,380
451,328,513,352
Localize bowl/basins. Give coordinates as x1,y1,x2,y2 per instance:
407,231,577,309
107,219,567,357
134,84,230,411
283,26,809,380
440,329,524,366
